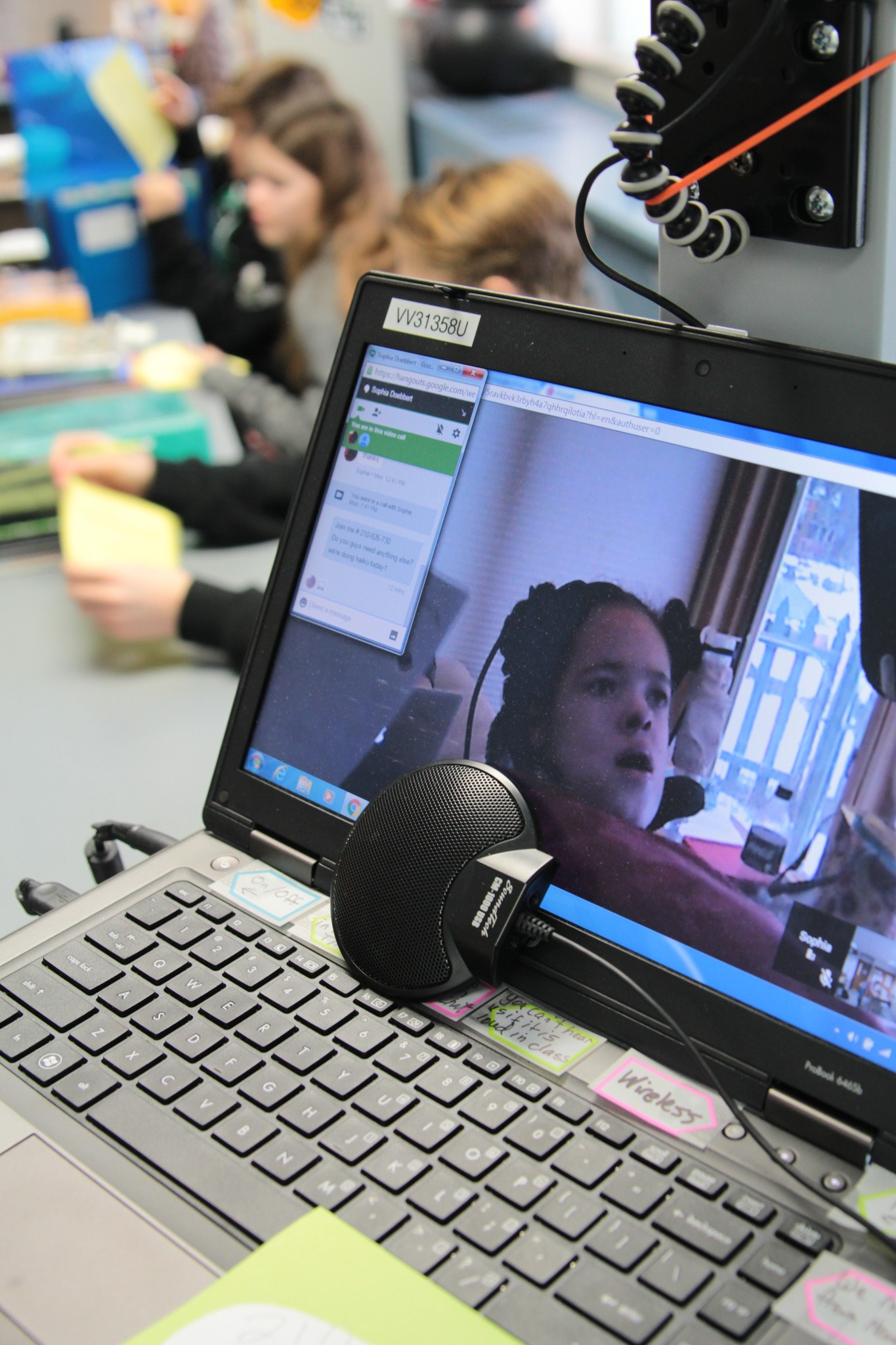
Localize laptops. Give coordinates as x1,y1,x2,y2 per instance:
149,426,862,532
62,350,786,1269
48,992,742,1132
0,272,896,1345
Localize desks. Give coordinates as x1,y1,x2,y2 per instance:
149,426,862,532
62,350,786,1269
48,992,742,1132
682,834,807,891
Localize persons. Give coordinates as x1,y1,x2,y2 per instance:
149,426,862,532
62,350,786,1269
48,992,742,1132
48,157,594,678
483,579,837,1029
126,67,388,456
